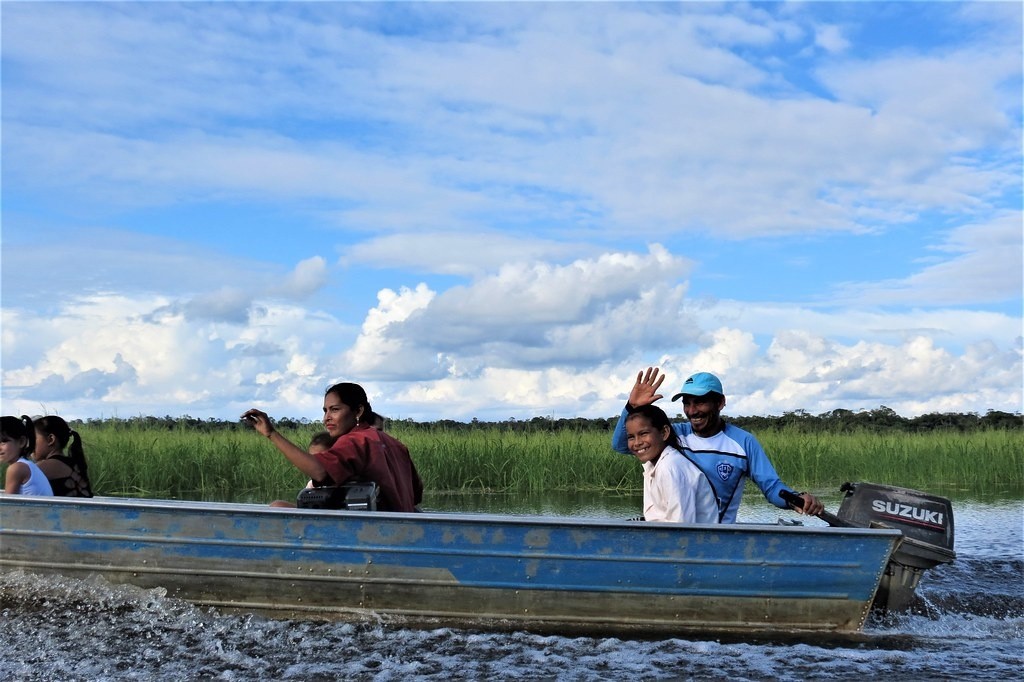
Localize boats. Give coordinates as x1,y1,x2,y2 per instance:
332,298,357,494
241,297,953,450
0,483,957,640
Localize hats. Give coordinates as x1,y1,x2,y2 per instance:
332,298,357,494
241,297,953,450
671,372,723,402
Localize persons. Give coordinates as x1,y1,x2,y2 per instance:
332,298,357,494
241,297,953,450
33,416,94,498
625,405,719,524
0,415,54,496
612,367,825,524
266,432,337,508
241,383,423,512
